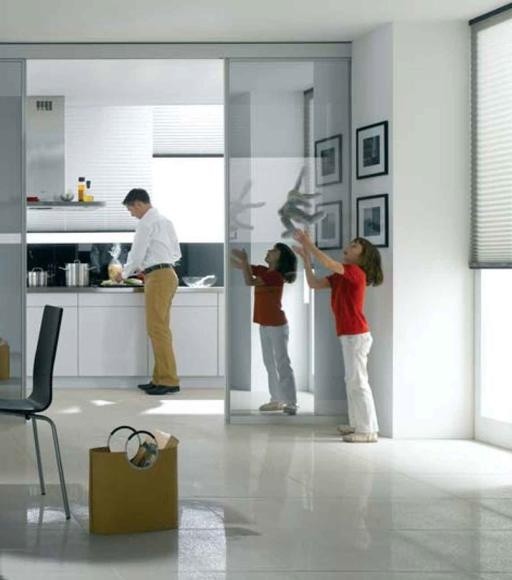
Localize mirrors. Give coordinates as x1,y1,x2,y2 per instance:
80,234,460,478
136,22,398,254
224,55,354,423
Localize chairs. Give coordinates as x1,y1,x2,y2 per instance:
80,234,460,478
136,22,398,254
0,305,72,519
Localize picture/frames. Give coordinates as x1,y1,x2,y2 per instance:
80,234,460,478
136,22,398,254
355,121,388,178
314,200,342,250
315,134,341,186
357,194,388,248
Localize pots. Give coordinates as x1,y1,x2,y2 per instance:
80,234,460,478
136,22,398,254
59,259,97,287
28,267,48,286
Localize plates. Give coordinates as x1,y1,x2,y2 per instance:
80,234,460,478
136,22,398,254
181,275,216,288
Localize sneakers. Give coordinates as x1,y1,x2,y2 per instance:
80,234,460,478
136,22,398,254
283,403,297,414
260,402,285,410
336,423,356,433
343,433,378,442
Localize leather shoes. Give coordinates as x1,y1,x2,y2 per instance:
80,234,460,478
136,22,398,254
138,382,157,390
143,263,172,274
146,385,180,395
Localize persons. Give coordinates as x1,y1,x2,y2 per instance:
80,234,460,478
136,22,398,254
114,189,183,395
291,227,384,443
232,243,297,416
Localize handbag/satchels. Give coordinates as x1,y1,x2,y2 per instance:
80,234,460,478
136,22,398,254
88,426,178,534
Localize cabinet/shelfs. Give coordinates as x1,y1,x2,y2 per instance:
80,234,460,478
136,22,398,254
78,289,219,391
24,290,79,386
